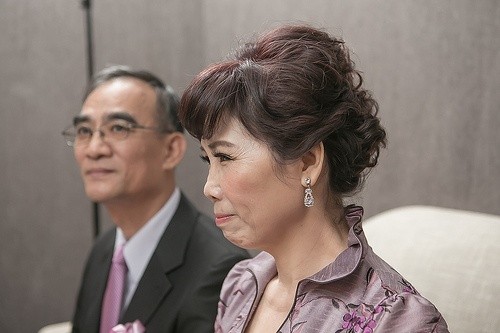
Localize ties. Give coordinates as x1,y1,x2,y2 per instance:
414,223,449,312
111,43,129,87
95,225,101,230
98,247,124,332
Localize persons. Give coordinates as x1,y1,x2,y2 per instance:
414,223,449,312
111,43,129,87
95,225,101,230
172,24,454,333
70,59,251,333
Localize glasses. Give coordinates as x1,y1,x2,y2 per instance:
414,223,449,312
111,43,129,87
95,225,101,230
61,120,169,146
